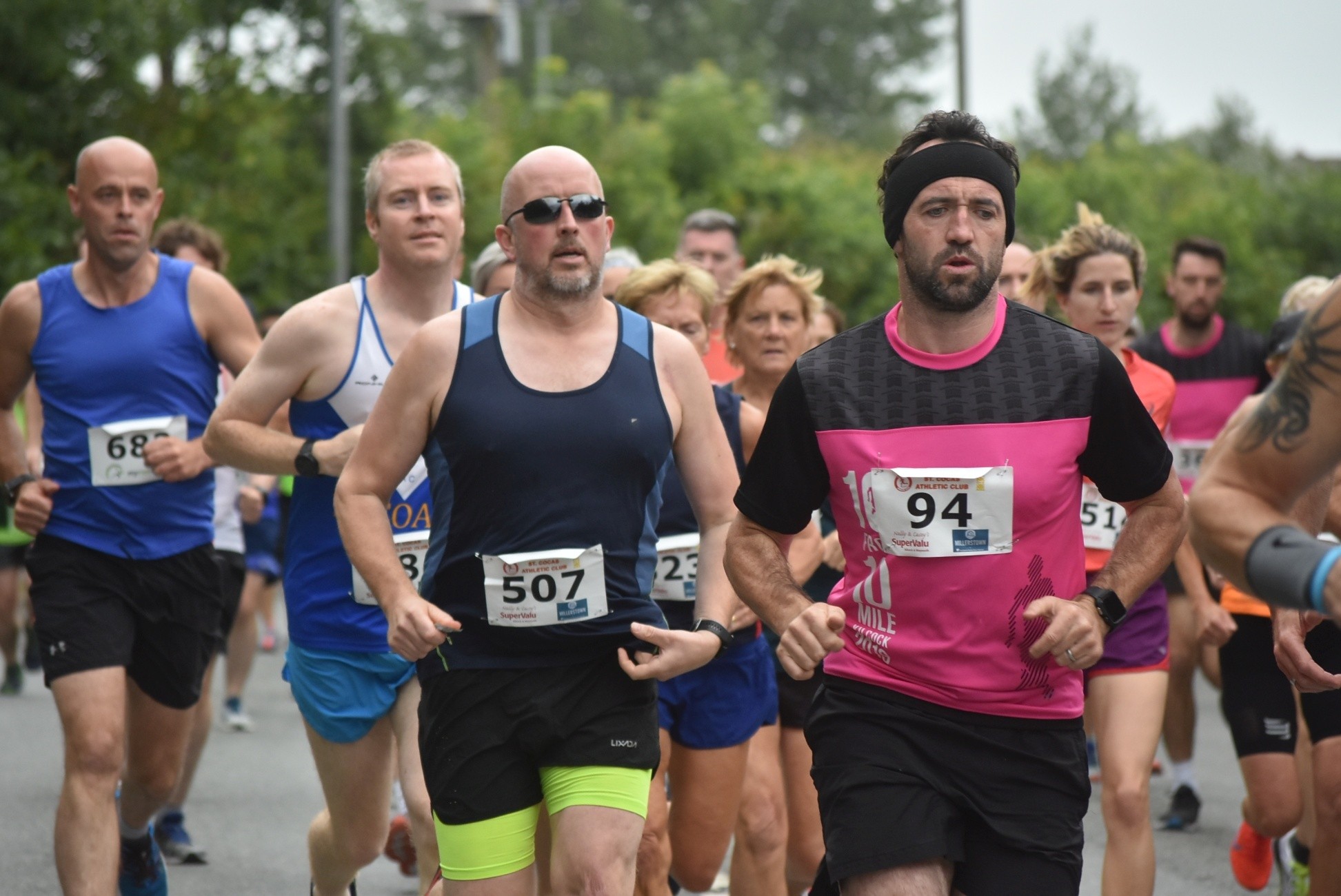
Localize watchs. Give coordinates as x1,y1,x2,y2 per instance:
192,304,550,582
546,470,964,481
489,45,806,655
294,437,320,480
1079,585,1128,634
0,473,37,508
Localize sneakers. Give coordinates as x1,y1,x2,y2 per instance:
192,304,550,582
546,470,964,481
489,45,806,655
118,824,171,896
158,810,206,866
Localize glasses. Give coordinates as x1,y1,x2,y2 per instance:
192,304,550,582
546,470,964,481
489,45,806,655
506,193,608,224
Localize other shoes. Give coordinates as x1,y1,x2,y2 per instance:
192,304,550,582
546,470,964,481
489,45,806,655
1230,819,1273,892
1275,827,1311,896
385,814,418,878
221,702,251,732
24,626,42,671
1156,783,1202,831
1,664,24,693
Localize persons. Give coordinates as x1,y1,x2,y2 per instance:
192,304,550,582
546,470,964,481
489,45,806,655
0,135,268,895
331,143,746,895
0,207,1341,896
719,107,1194,896
198,135,490,895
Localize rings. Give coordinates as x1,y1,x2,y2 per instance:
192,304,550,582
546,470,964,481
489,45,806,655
1290,678,1297,686
1066,648,1078,664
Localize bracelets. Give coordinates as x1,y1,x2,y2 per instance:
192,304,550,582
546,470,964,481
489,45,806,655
689,618,735,650
1238,522,1337,611
